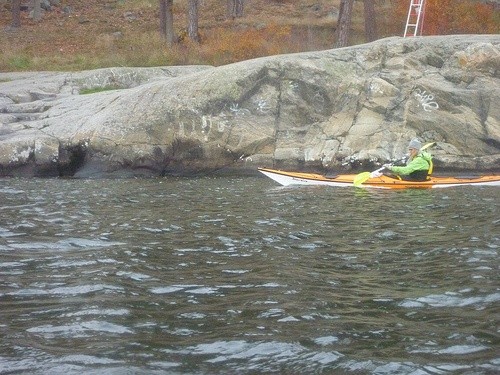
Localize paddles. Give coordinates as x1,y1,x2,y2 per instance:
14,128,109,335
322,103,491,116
353,142,437,185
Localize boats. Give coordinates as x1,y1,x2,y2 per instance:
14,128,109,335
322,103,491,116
257,167,500,187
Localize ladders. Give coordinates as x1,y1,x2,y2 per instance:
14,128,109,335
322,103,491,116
403,0,426,38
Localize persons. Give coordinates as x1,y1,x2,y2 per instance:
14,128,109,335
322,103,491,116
382,140,430,182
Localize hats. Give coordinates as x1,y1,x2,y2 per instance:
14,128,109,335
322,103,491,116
408,138,421,151
